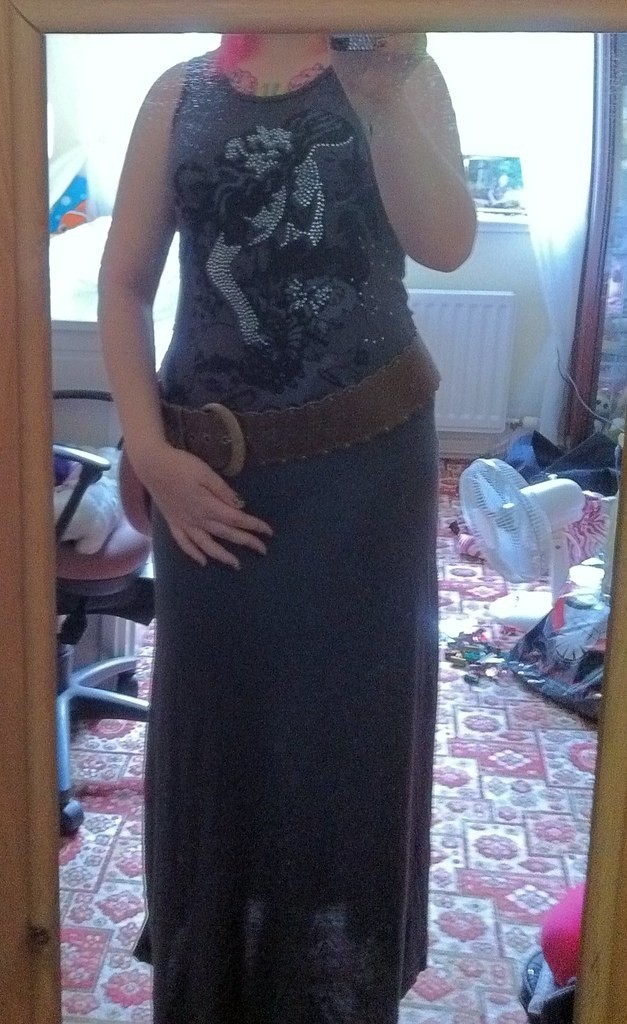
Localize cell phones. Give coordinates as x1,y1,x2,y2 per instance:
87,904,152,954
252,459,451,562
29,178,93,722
328,32,388,51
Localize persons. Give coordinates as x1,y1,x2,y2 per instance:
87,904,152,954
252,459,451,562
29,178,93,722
97,32,478,1024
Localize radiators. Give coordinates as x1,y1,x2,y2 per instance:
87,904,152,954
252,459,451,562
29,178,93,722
404,289,536,434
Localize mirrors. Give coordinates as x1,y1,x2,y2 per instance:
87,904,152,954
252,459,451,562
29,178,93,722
0,0,627,1024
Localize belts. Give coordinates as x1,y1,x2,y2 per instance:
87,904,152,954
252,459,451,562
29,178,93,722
158,337,442,482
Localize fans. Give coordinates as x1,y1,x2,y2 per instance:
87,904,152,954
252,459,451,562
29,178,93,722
460,456,585,627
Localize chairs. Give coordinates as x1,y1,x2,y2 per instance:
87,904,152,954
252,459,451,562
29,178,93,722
53,388,156,836
